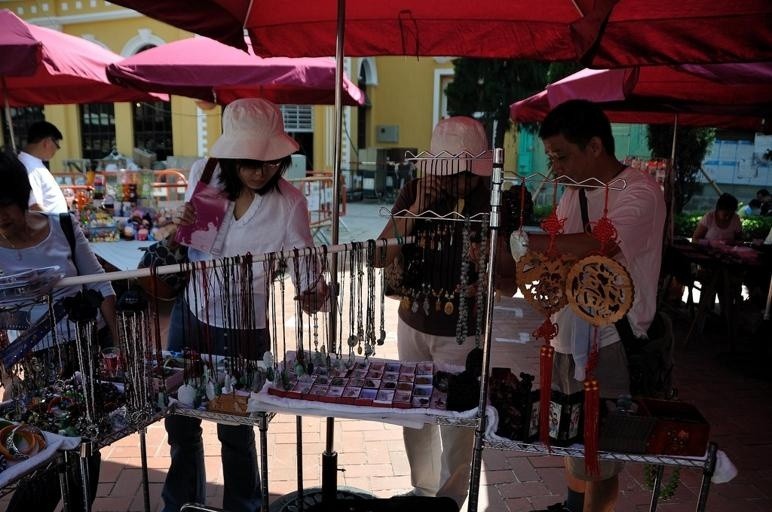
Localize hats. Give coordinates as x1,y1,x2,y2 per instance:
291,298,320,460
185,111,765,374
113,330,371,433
414,116,493,177
209,98,300,161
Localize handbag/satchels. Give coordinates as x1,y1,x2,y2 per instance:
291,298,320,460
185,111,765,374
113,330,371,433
626,336,678,400
136,232,191,305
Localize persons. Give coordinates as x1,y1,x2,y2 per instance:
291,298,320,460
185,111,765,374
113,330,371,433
364,113,515,512
737,198,762,217
155,93,340,512
16,119,71,216
760,195,772,217
750,222,772,248
690,192,744,317
756,188,771,207
0,147,132,512
510,95,671,512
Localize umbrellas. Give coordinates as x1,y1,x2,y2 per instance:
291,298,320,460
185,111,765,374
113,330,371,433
105,0,772,512
0,7,179,159
507,52,772,232
104,27,367,131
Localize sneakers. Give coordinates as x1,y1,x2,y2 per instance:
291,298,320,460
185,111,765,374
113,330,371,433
529,500,572,512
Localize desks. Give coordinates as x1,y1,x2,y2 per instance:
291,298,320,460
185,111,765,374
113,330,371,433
1,353,224,511
88,240,159,293
479,394,738,511
672,251,772,352
160,364,303,511
254,352,481,510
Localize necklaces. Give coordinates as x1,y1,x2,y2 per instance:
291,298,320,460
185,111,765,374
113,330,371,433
0,214,490,474
417,158,476,253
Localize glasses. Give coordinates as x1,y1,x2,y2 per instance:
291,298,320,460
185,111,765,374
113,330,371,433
240,162,283,177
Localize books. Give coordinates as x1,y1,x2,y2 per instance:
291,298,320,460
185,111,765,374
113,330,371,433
170,181,236,255
